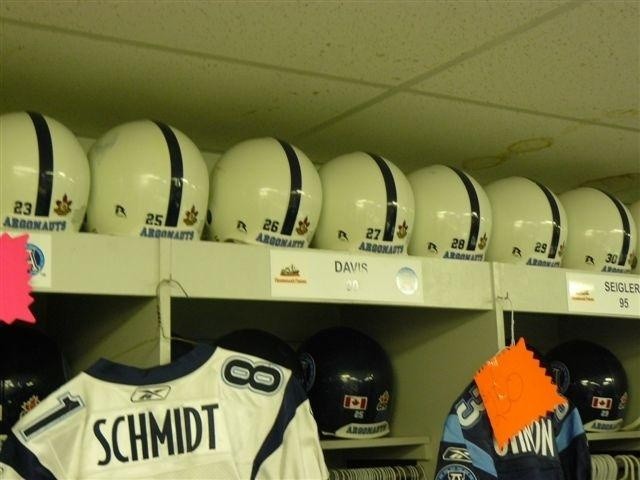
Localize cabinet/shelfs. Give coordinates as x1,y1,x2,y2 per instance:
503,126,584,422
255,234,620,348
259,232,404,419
0,229,640,480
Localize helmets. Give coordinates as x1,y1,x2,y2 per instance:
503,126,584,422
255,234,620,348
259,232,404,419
82,119,210,241
202,135,324,249
308,150,416,255
0,110,91,233
482,175,568,268
296,326,395,441
557,186,638,274
0,323,73,434
406,163,493,262
543,339,630,434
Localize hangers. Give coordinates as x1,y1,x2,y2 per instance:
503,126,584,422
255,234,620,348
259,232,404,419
324,462,429,480
96,275,209,374
589,454,640,480
